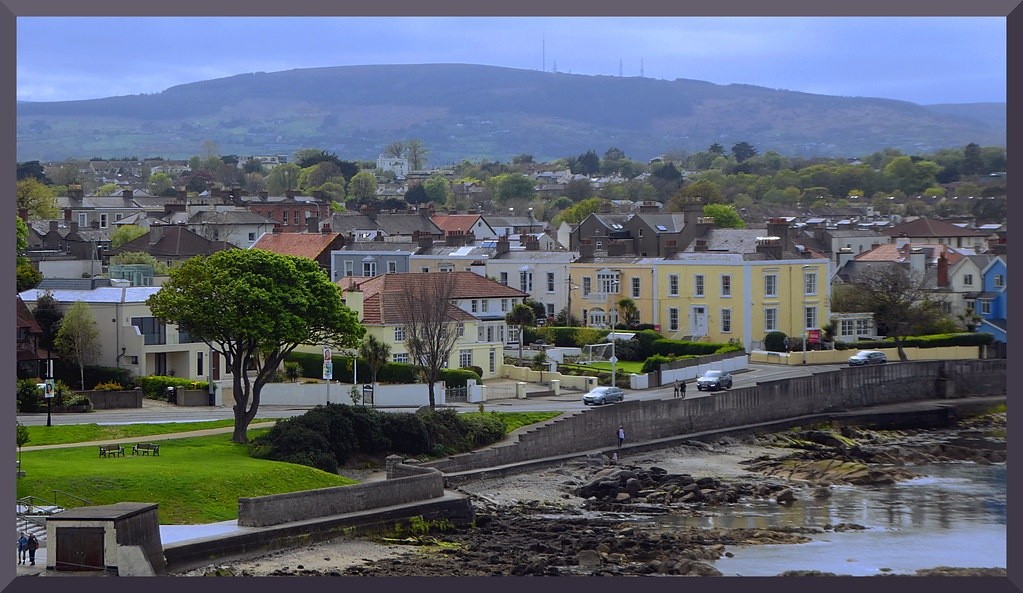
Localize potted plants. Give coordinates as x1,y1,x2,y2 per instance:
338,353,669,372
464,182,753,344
63,394,90,412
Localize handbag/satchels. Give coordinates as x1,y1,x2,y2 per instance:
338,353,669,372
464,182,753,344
36,543,39,548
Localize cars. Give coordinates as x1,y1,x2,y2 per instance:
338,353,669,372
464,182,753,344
847,349,887,366
697,370,733,390
582,386,624,407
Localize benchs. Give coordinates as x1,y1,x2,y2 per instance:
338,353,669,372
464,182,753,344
98,444,125,458
132,442,160,456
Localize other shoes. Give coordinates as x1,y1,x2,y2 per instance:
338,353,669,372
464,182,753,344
18,562,21,565
23,561,25,565
30,562,35,566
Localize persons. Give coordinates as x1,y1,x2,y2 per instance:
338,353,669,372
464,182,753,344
18,532,38,566
46,384,53,394
616,425,625,447
324,350,331,360
613,449,619,465
673,379,687,398
